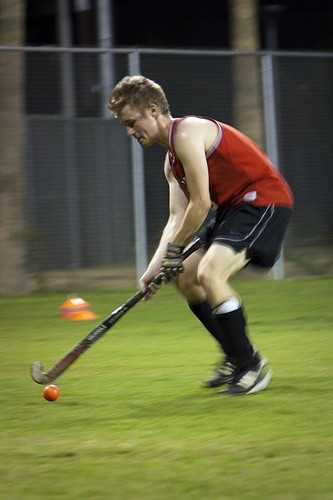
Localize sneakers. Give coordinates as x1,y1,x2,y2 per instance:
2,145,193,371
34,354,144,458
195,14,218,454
204,359,238,388
218,352,273,395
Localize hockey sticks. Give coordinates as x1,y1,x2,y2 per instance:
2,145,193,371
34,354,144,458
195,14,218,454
31,237,200,384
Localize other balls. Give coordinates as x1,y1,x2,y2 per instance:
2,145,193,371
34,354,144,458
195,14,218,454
43,384,60,402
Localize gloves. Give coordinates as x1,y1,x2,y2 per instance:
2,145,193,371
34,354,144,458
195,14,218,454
160,241,185,284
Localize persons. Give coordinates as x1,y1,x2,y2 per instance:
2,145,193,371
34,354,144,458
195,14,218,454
106,75,295,396
